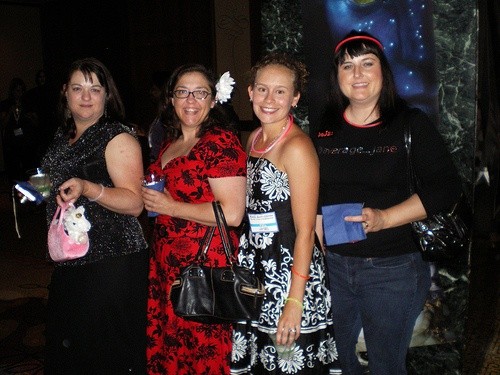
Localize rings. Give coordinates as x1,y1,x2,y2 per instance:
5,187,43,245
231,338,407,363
289,329,297,332
364,222,368,227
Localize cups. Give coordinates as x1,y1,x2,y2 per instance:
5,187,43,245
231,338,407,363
143,176,167,218
30,175,51,197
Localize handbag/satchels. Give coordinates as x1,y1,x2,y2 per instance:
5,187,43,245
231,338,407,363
169,200,265,324
47,201,91,262
403,109,471,261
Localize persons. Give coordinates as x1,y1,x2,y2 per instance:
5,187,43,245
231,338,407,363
229,49,343,375
141,64,247,375
302,28,459,375
17,60,143,375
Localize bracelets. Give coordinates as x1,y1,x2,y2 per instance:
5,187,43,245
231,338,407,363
88,183,104,202
291,266,310,280
284,298,303,312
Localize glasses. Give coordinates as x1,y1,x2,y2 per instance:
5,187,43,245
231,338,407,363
171,90,212,99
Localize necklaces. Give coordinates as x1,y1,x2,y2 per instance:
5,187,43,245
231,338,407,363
247,112,293,179
